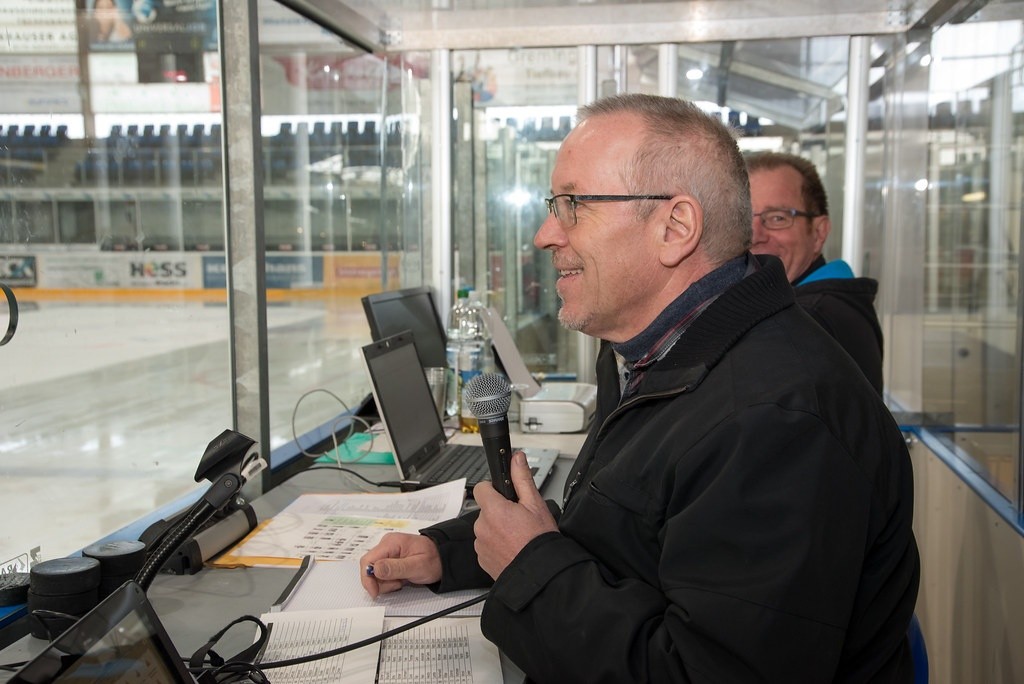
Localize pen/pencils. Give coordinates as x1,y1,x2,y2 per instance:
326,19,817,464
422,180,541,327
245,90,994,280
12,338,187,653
365,565,375,576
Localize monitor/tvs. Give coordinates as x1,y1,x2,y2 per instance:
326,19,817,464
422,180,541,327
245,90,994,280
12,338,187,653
361,285,448,377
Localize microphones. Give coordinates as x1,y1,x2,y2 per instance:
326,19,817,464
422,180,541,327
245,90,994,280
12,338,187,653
465,374,519,504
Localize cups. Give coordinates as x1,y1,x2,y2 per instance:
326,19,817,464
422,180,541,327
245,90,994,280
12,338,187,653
424,367,448,425
507,384,528,421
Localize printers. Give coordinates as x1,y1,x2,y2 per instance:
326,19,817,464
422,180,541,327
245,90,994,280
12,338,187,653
491,344,599,433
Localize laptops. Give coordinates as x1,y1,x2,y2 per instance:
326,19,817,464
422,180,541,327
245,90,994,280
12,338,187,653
6,580,195,684
361,328,559,495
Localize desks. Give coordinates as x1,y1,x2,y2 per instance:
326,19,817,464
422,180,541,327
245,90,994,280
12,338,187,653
148,422,594,684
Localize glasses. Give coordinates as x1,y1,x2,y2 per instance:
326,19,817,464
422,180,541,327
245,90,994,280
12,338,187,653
753,207,818,231
545,189,675,230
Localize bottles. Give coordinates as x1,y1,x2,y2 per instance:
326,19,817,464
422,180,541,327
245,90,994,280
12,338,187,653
446,287,495,433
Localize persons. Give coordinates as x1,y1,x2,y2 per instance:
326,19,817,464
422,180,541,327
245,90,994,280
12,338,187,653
85,1,132,46
742,148,884,399
361,94,920,684
